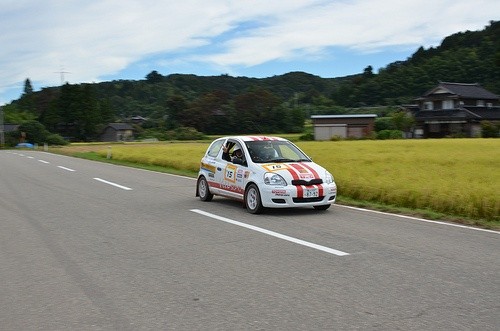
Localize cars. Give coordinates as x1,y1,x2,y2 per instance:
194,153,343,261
196,136,338,214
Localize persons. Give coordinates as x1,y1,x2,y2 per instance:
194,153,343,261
221,141,243,162
